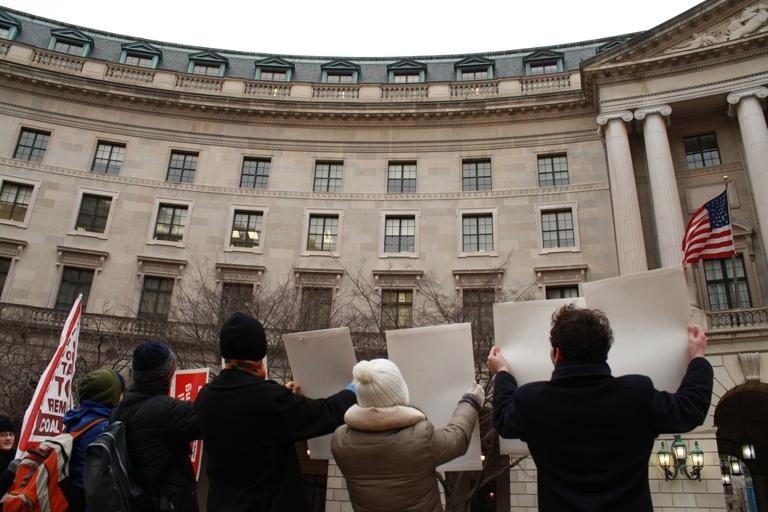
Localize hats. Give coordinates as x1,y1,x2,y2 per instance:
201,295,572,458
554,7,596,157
220,311,267,360
352,358,410,408
130,343,178,385
0,415,14,432
79,367,123,405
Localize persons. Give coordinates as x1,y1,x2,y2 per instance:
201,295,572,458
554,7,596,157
109,340,207,512
0,415,15,511
62,368,125,512
193,312,358,512
486,303,713,512
331,358,486,512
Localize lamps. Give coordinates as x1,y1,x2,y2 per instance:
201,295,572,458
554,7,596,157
658,435,704,482
721,418,756,486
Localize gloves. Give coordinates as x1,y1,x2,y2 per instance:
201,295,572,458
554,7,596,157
466,383,485,409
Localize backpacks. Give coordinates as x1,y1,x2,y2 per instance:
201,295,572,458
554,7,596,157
0,418,111,512
82,397,188,512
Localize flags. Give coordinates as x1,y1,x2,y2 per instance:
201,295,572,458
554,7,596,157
682,189,737,271
15,293,83,462
